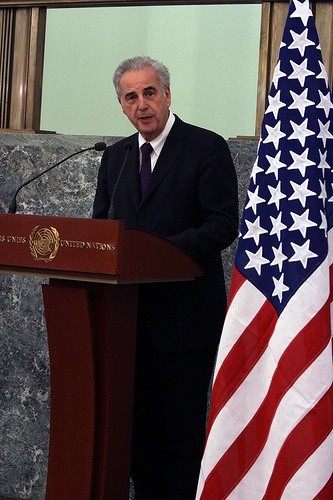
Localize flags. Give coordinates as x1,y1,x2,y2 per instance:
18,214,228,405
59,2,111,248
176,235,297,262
195,0,333,500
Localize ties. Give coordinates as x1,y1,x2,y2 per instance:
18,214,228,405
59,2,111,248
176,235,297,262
140,142,154,203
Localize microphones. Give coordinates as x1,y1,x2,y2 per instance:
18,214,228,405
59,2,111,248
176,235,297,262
9,142,106,214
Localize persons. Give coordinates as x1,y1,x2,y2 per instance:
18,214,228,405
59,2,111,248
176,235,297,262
93,56,238,500
